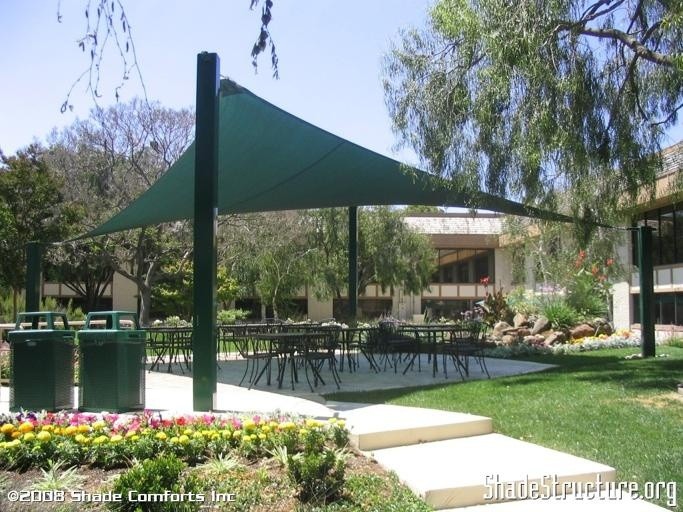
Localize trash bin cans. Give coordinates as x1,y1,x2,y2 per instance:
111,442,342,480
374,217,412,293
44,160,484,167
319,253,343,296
78,311,147,413
8,311,75,412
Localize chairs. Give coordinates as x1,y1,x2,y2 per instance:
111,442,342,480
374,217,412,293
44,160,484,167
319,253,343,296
141,323,492,394
